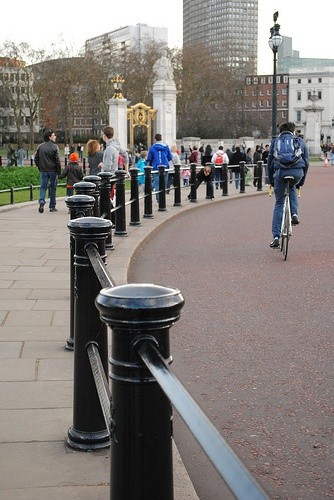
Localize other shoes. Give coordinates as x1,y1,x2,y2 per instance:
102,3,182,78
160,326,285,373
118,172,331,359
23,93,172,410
50,208,58,212
38,203,44,213
270,236,279,248
292,214,300,225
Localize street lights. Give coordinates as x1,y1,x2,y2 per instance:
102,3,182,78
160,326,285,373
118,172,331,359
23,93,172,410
267,10,283,142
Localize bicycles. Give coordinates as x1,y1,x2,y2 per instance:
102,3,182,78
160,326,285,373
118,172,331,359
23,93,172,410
269,175,302,260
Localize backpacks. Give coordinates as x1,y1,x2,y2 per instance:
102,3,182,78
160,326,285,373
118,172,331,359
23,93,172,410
270,134,303,169
215,152,225,165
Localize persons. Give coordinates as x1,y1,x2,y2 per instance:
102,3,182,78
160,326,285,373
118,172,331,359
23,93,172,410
5,141,26,167
98,126,121,210
64,142,85,163
152,48,176,81
118,141,270,201
57,150,84,215
34,130,62,214
266,122,310,249
319,142,334,167
146,133,175,203
87,138,104,175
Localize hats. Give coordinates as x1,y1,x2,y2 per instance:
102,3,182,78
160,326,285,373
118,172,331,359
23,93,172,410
69,152,78,160
